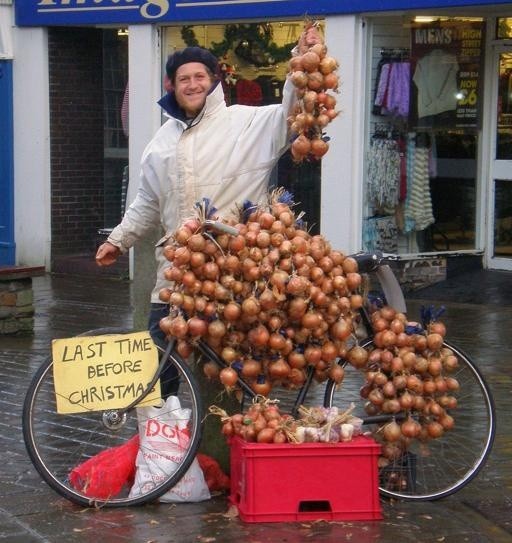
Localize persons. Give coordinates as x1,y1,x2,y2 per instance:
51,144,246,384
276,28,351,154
95,20,327,408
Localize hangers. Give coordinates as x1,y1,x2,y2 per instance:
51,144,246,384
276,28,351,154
379,46,411,61
372,121,430,137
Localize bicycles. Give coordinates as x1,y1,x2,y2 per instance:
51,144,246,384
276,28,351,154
23,219,496,508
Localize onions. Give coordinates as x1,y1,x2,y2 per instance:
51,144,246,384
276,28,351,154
159,201,459,459
288,44,340,164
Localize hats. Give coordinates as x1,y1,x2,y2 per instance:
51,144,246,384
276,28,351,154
164,45,222,83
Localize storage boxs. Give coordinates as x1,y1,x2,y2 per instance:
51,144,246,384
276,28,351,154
228,430,385,522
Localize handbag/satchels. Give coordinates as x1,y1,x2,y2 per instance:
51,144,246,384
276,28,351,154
124,392,214,506
